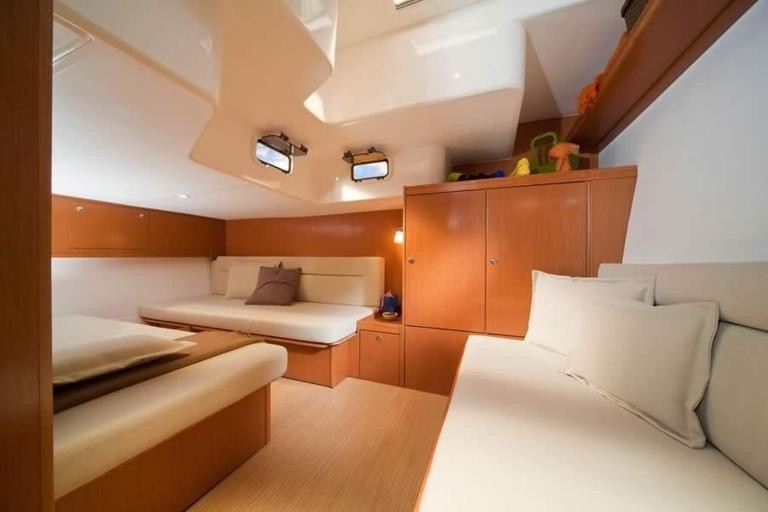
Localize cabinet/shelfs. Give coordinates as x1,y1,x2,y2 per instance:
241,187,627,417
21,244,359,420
354,316,399,386
401,164,637,397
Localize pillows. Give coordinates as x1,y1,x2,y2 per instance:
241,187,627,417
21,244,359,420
52,333,186,386
556,291,719,449
223,262,280,299
524,269,655,355
243,265,302,304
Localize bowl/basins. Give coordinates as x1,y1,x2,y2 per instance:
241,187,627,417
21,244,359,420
382,311,398,321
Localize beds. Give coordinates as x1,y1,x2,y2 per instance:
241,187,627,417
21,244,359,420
51,311,288,512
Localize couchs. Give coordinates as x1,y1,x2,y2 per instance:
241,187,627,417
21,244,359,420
138,255,381,387
415,260,768,512
